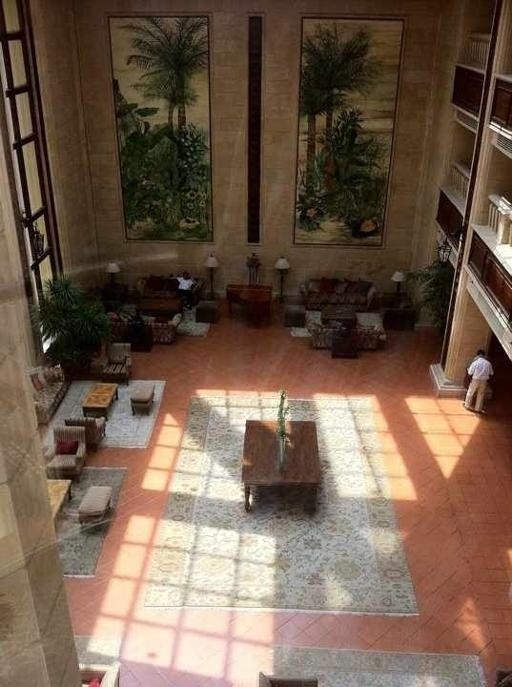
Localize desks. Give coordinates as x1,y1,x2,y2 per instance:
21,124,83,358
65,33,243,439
242,419,320,513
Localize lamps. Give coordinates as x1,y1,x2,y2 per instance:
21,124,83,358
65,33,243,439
104,262,121,295
202,254,220,299
391,268,407,303
273,255,291,296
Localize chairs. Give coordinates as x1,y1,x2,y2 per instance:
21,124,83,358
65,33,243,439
331,329,358,358
43,416,106,483
99,342,133,387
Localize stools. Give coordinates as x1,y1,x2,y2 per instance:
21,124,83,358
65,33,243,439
130,382,155,415
194,299,221,326
79,485,113,530
284,303,306,328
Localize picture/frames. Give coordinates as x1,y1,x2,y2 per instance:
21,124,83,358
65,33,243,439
104,9,216,246
294,16,408,255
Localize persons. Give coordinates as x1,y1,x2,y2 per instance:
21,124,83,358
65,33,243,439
160,271,196,306
463,348,495,416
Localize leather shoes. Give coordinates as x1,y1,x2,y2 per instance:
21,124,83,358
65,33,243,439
185,305,192,310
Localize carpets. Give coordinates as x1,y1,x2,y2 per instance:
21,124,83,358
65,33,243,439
142,395,420,619
50,377,166,449
55,464,128,579
74,633,119,669
290,323,310,339
177,307,214,338
269,644,488,687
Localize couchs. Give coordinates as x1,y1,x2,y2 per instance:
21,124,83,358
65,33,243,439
297,278,382,313
131,274,208,313
107,310,182,344
27,363,72,425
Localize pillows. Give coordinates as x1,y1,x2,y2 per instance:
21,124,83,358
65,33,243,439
31,364,64,391
144,274,179,294
